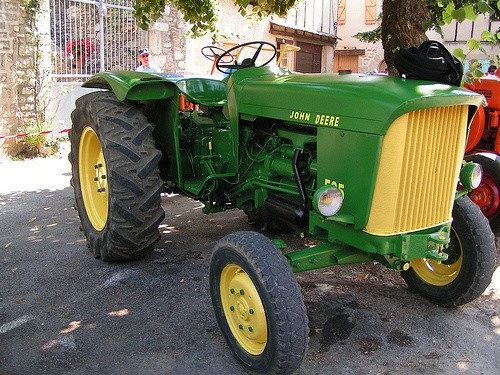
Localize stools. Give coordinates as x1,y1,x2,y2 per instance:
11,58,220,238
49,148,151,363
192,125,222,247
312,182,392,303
177,77,231,117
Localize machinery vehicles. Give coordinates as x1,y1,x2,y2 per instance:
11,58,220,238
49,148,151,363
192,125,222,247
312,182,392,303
434,64,500,229
68,40,496,374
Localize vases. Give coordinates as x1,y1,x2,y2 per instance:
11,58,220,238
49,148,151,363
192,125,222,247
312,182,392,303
75,58,85,69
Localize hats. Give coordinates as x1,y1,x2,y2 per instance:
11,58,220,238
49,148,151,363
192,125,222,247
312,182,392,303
138,48,150,55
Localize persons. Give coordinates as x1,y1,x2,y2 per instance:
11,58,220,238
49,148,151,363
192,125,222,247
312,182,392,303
466,63,498,77
134,48,162,74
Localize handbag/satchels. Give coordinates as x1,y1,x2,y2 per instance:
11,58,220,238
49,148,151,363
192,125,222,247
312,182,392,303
395,40,463,87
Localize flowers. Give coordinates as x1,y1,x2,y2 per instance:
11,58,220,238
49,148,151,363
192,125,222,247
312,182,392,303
64,37,95,62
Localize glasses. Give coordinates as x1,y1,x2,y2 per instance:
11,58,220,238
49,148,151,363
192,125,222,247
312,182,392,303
488,70,494,72
